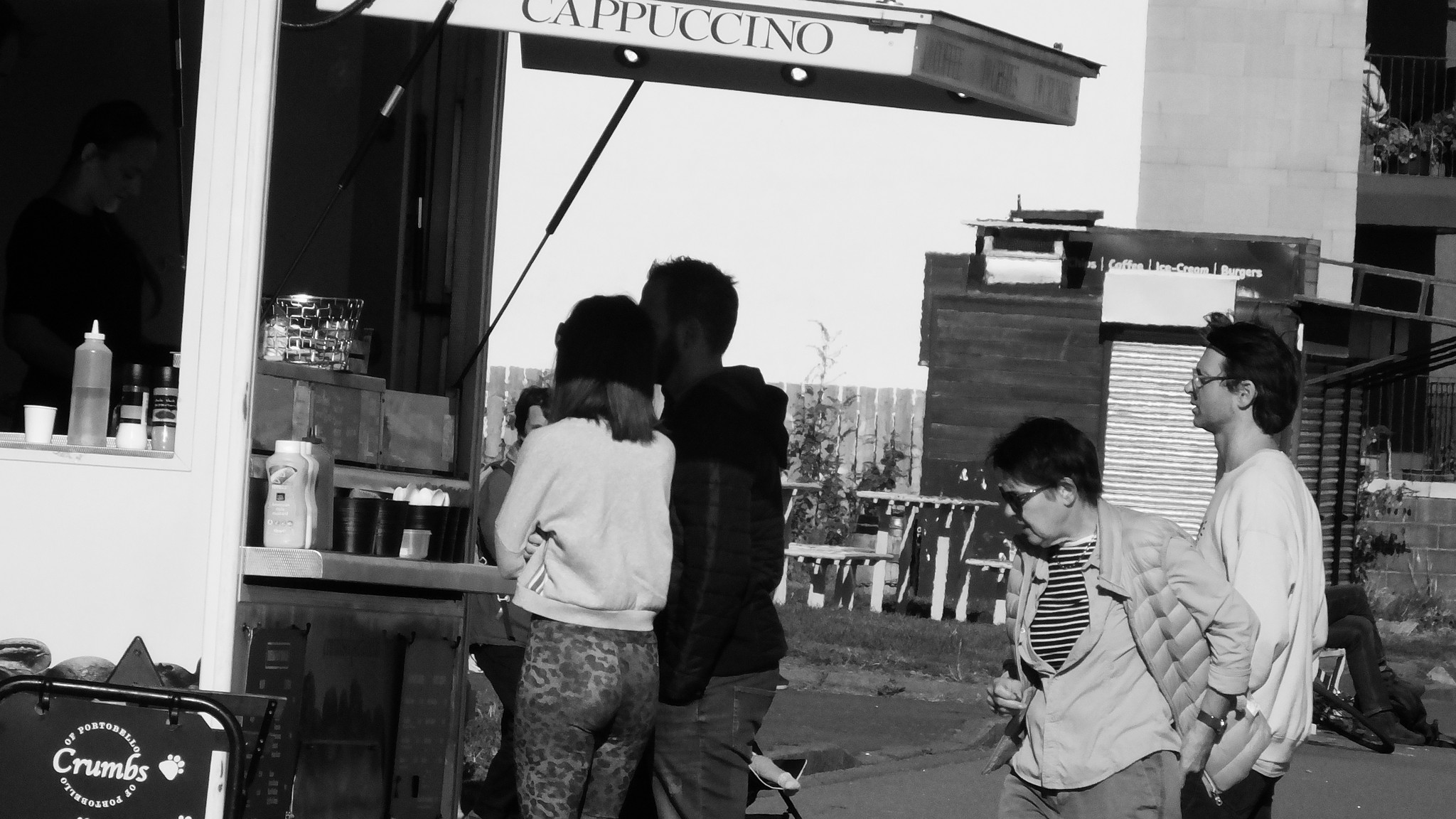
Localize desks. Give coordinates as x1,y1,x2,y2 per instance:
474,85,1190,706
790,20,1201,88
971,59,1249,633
783,539,894,610
964,556,1014,625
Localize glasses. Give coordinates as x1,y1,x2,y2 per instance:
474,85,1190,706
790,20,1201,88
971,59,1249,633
1192,372,1247,390
1004,482,1056,513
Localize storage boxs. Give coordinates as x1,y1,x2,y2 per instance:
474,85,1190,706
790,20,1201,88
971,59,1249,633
252,365,460,474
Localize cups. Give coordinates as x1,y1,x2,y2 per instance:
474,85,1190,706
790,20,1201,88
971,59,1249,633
407,504,470,565
371,500,410,557
332,497,379,555
24,404,57,444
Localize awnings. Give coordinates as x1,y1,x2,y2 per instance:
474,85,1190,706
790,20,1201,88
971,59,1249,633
313,0,1101,131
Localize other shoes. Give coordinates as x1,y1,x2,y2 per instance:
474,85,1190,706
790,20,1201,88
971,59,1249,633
1352,706,1426,746
1380,666,1425,694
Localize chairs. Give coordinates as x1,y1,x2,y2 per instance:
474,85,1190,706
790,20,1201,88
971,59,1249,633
748,738,815,819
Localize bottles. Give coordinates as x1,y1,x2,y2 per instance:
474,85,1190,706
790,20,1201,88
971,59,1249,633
115,363,151,450
151,367,178,452
263,427,334,551
67,319,112,448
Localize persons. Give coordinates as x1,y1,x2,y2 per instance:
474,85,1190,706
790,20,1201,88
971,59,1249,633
1,99,169,432
477,255,798,819
1324,584,1426,745
983,414,1259,819
1188,311,1330,819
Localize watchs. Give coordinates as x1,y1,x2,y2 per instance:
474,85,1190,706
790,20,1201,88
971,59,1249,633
1198,712,1227,737
